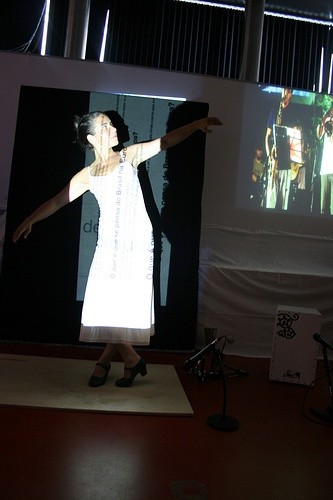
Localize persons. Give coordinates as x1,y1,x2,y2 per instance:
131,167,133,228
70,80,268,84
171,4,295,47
13,111,224,389
250,88,332,215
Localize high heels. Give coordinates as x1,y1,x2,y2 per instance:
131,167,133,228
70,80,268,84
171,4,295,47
115,358,148,387
89,362,112,387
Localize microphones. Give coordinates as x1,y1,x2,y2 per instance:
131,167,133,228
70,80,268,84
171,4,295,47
313,333,333,351
184,337,224,370
